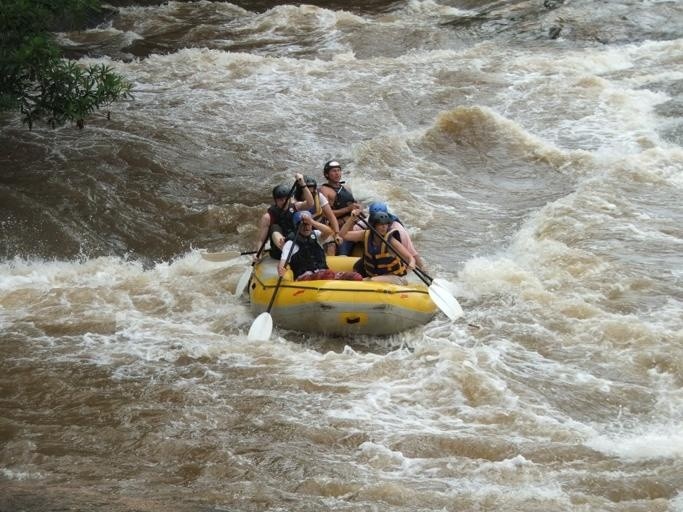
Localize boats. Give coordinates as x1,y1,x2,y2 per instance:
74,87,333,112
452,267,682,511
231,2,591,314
247,241,439,337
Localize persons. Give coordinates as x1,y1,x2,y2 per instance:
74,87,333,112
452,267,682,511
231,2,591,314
252,159,429,286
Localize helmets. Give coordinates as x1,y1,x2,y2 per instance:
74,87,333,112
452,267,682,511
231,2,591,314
372,212,389,224
292,210,312,229
369,202,386,215
324,161,341,174
273,185,290,199
296,176,317,190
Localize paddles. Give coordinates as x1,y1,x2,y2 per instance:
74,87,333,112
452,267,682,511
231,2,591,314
200,248,272,262
248,221,304,340
236,178,301,297
355,220,457,297
354,211,463,319
